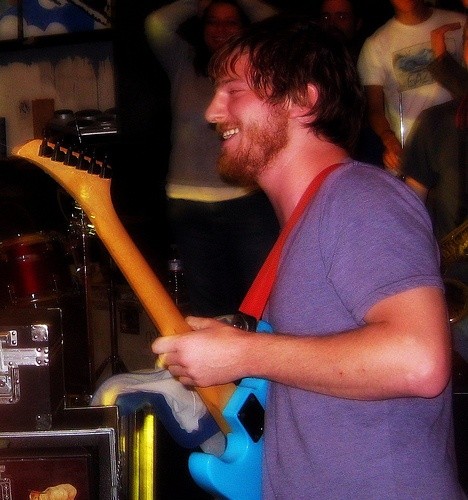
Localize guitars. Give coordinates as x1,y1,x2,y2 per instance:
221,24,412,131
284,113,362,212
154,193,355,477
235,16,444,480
11,126,274,500
438,219,468,322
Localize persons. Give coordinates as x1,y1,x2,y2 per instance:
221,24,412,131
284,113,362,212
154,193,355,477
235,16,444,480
145,0,468,500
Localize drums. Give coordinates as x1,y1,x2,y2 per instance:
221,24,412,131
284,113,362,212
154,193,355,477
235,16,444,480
0,229,75,308
